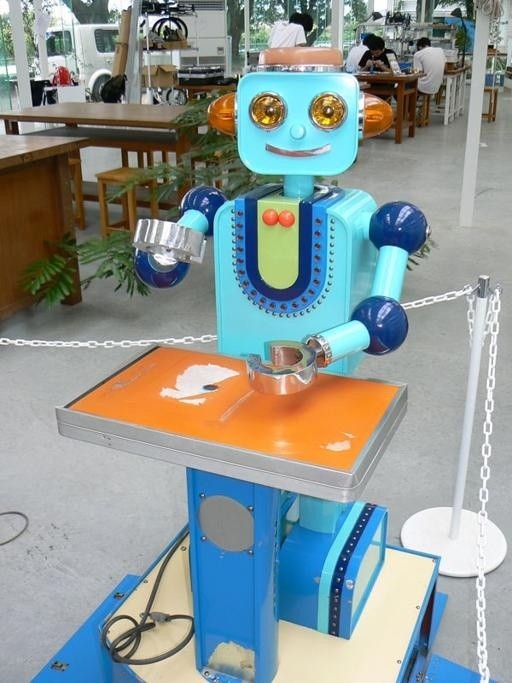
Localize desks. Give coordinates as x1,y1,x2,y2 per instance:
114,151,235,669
0,101,225,325
178,83,236,104
344,59,472,145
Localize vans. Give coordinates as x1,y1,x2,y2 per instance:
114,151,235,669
32,23,145,103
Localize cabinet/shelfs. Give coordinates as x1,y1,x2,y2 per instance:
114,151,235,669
135,9,200,107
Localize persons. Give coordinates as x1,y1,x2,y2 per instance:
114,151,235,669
268,13,324,46
411,36,447,96
344,32,376,73
124,46,434,639
359,37,399,101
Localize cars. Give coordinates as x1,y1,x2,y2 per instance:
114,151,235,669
238,44,259,53
0,56,36,84
316,27,401,48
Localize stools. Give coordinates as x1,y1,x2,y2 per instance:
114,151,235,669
95,165,158,244
67,157,86,230
120,147,170,184
481,87,498,123
